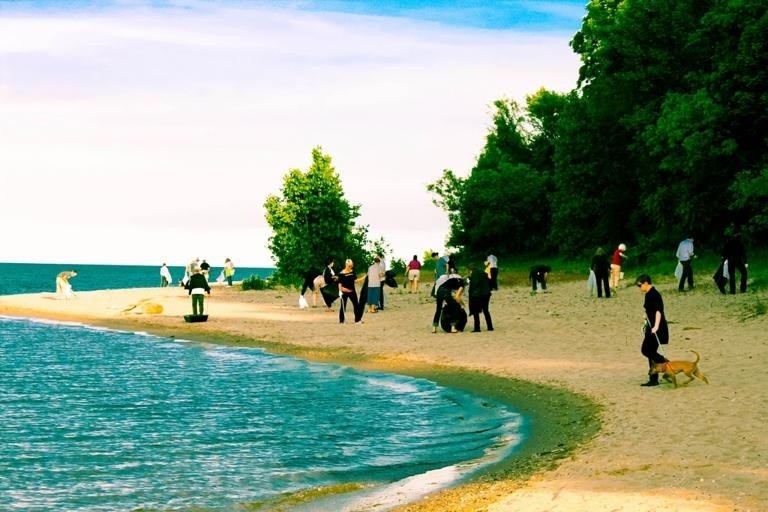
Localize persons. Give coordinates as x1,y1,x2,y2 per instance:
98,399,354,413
636,275,669,386
721,236,749,294
189,269,211,316
675,235,698,292
591,247,612,298
609,243,628,288
529,265,551,293
301,253,499,333
181,257,211,287
160,263,172,288
223,258,234,287
56,270,77,300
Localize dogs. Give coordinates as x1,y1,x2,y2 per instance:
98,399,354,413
648,349,710,390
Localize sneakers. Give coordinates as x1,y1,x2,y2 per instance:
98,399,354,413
640,379,660,386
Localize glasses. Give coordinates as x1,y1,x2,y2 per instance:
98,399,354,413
637,283,642,287
